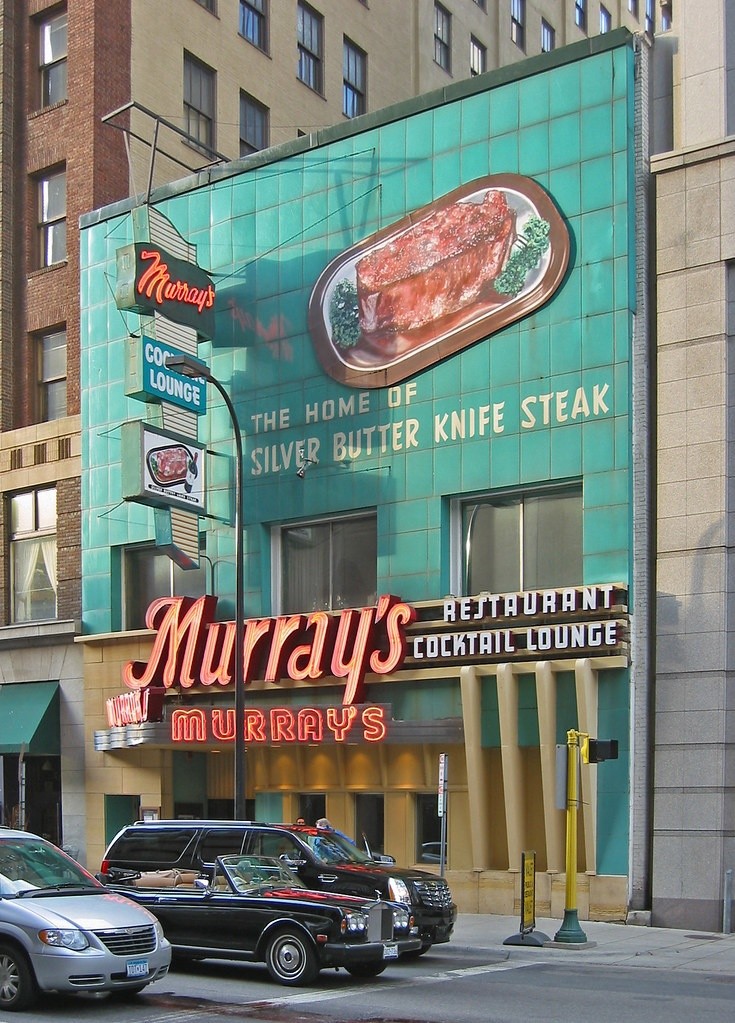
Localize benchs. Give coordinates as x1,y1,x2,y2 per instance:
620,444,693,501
132,871,176,887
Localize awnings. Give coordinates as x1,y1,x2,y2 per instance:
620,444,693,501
0,681,60,753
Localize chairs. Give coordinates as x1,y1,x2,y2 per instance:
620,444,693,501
174,873,197,888
212,876,228,891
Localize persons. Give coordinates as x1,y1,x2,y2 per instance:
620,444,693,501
296,817,304,824
315,818,355,866
226,861,278,891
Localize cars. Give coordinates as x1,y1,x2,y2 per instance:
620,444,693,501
0,828,171,1011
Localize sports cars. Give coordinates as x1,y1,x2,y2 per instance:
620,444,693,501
90,854,421,986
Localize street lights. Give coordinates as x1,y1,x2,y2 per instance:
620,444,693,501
164,355,245,817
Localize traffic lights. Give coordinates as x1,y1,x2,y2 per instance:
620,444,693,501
582,735,618,764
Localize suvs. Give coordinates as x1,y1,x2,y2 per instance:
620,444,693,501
99,819,457,959
